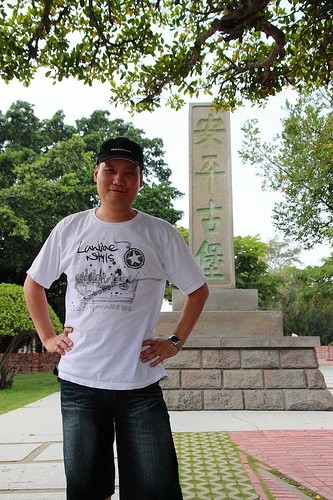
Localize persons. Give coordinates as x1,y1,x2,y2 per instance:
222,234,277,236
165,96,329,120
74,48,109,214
23,137,209,500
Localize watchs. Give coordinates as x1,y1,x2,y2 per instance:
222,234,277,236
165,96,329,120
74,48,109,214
167,335,182,352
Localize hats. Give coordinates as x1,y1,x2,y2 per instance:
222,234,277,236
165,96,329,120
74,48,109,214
96,136,145,170
